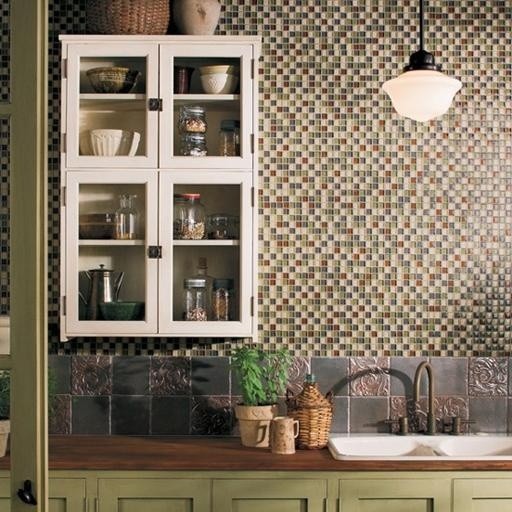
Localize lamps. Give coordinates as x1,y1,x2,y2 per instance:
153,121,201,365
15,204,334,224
381,0,462,123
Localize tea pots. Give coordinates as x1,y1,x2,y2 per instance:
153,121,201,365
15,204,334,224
78,263,127,321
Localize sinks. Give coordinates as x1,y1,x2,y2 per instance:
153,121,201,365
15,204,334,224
428,433,512,461
327,432,440,460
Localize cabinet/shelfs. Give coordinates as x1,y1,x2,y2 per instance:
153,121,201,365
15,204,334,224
48,477,88,512
95,476,327,512
65,171,253,335
337,477,512,512
66,42,253,169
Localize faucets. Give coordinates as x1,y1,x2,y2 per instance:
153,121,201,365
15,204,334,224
413,360,439,435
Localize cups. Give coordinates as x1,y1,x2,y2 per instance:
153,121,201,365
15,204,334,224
269,417,301,455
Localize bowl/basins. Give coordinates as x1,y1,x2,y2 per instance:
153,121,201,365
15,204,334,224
85,66,142,92
79,213,114,239
197,64,239,94
98,299,144,321
79,127,141,156
173,65,196,93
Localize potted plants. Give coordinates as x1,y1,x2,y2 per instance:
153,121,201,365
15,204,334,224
0,368,11,457
228,343,293,448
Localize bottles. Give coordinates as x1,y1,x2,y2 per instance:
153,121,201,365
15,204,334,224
114,194,138,242
174,192,206,239
177,107,208,156
186,259,216,307
181,279,208,321
209,278,236,321
217,120,239,156
206,212,239,239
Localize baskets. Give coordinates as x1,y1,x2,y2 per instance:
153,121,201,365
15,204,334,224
86,0,169,35
286,384,334,449
